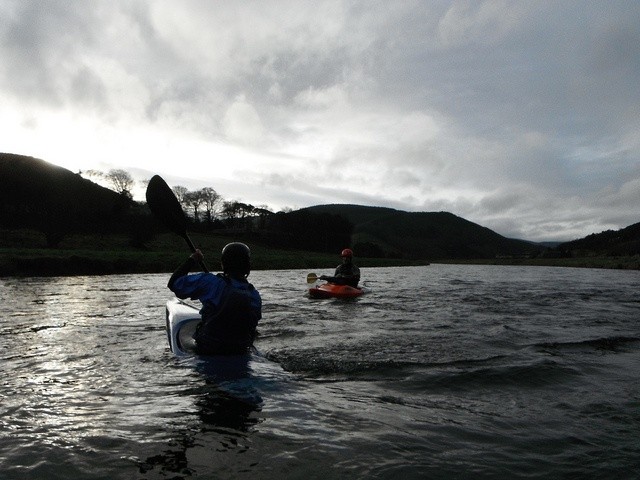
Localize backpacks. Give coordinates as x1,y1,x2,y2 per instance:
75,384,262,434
193,273,253,352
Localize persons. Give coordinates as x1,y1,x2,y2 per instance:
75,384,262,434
319,249,361,288
166,241,262,358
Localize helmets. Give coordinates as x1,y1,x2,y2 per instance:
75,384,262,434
221,242,251,266
342,249,353,256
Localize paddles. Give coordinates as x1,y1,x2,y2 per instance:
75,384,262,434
307,273,352,283
146,175,258,273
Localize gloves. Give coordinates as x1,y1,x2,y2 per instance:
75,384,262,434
336,273,342,279
319,276,327,280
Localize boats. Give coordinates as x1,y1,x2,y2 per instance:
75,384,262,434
309,284,372,297
165,297,258,355
